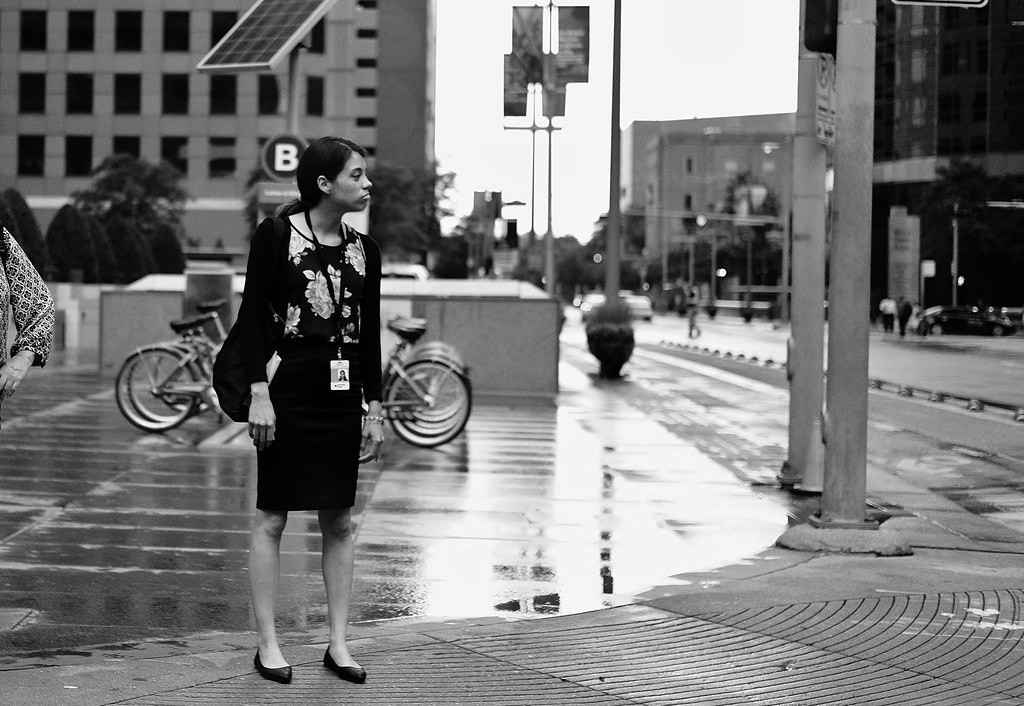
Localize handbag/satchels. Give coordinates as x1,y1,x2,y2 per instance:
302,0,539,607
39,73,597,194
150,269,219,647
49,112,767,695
211,296,269,423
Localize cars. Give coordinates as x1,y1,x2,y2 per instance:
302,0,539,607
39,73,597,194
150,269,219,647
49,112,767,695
910,305,1018,337
580,289,653,323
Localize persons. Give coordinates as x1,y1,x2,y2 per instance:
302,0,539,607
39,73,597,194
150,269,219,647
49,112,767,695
880,294,913,336
339,370,347,381
685,291,701,338
243,136,384,683
0,223,55,427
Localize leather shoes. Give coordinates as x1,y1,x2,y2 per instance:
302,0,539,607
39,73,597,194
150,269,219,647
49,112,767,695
323,645,367,684
254,648,292,684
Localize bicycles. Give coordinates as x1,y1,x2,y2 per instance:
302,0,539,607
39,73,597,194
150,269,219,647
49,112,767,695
114,298,474,447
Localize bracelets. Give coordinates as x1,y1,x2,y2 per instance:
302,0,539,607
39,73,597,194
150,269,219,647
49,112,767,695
364,417,385,422
